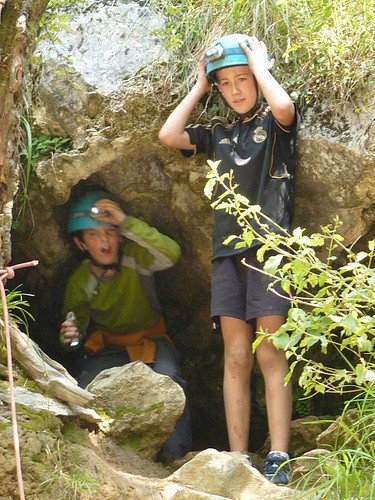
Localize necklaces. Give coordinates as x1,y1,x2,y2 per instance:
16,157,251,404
91,270,107,297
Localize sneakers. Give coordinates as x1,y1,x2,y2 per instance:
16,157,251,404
264,455,292,484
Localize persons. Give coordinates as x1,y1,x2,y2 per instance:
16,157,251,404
156,32,303,487
56,191,193,462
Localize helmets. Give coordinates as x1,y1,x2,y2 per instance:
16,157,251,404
65,190,123,230
206,34,268,77
